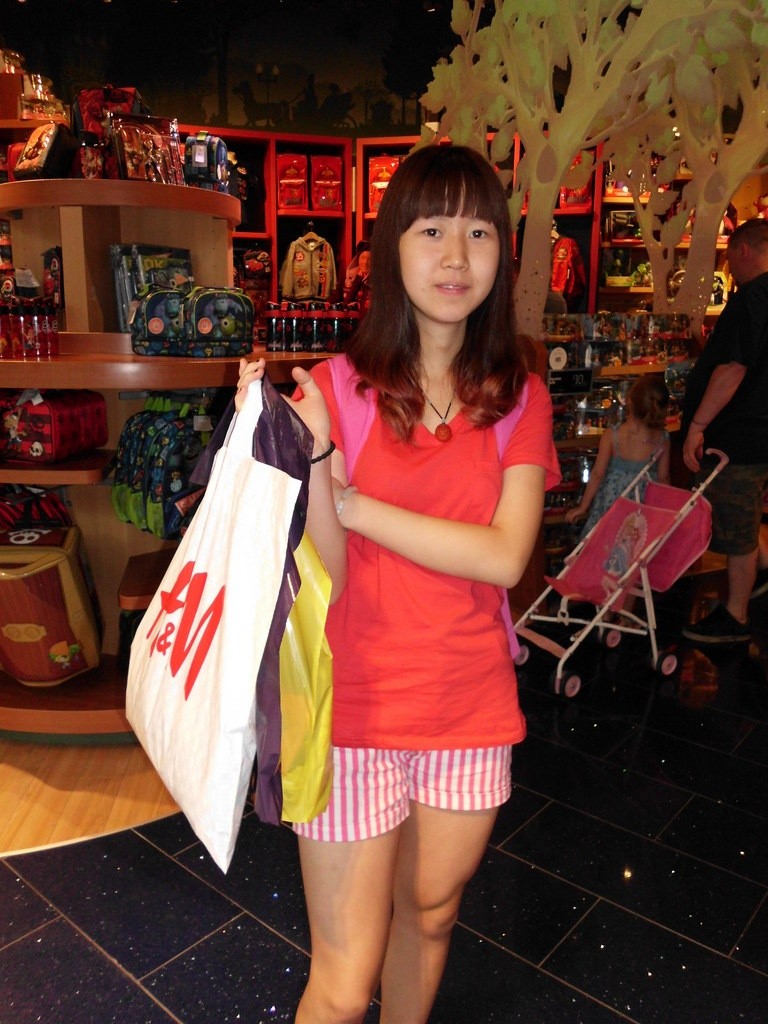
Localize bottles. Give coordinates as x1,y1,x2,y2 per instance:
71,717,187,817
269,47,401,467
662,367,684,405
267,301,358,352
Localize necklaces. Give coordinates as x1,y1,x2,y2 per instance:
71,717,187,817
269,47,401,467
420,384,456,443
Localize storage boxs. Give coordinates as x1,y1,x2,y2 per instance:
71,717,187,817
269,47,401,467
311,155,343,212
275,153,310,211
367,155,401,213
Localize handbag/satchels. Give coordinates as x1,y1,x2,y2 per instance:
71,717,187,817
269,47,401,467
252,377,333,824
125,374,294,873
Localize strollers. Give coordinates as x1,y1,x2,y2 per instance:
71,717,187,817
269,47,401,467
513,441,730,696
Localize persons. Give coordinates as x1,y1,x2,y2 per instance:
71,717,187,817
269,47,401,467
679,217,768,642
343,240,370,309
235,141,564,1024
564,375,671,629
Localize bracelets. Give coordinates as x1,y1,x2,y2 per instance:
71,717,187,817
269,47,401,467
311,442,336,464
335,485,358,513
692,420,708,425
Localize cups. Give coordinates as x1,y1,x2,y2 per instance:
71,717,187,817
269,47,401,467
0,316,59,359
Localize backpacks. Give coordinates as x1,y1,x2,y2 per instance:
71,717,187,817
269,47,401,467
14,121,73,178
230,153,249,202
111,397,218,539
129,283,185,354
179,284,254,356
183,130,228,191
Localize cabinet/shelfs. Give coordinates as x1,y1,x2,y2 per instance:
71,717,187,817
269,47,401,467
359,131,767,314
178,125,354,308
1,118,344,746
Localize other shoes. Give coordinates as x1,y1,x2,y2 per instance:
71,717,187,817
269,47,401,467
683,605,751,642
750,569,768,598
